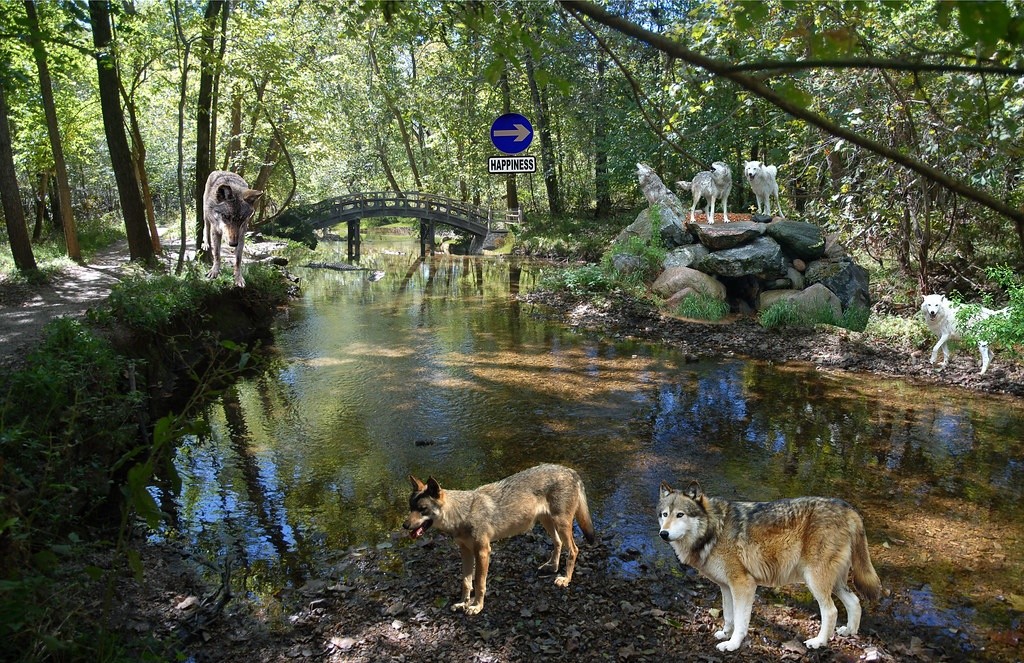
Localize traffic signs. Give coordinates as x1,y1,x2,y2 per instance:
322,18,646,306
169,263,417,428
488,110,537,173
